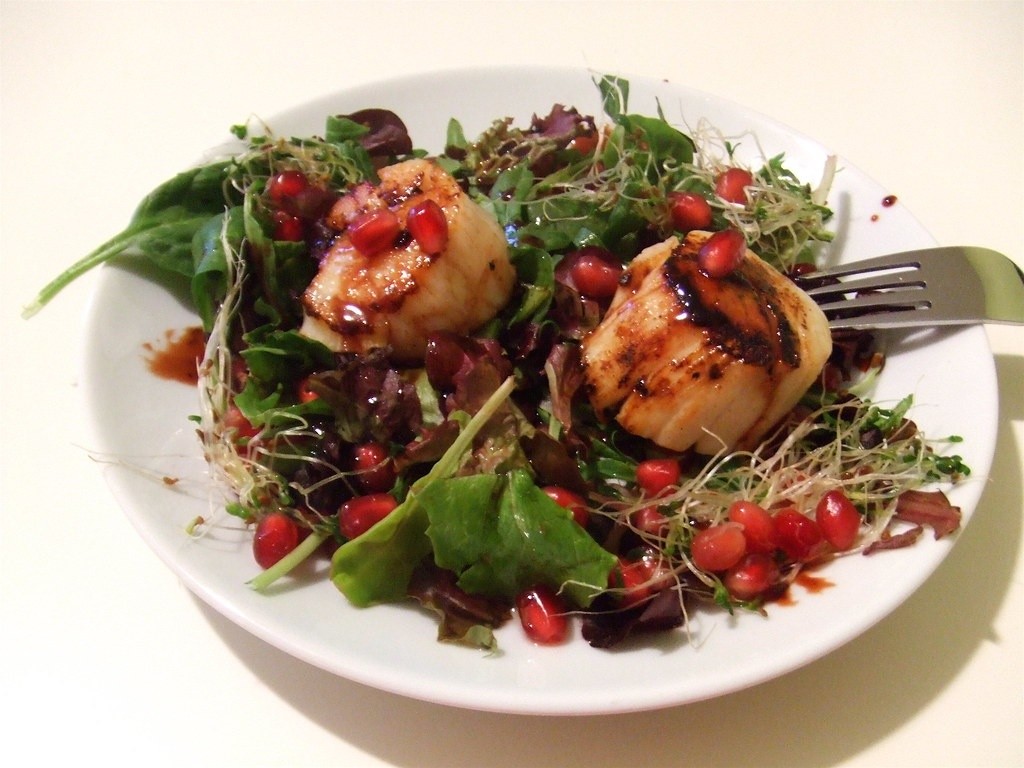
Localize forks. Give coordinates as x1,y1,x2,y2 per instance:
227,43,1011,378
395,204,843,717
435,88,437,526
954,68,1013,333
798,247,1024,329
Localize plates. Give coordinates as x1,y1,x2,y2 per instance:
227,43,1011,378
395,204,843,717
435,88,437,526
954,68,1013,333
84,63,997,716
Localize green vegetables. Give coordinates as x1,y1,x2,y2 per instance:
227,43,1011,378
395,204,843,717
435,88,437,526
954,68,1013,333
21,71,969,647
